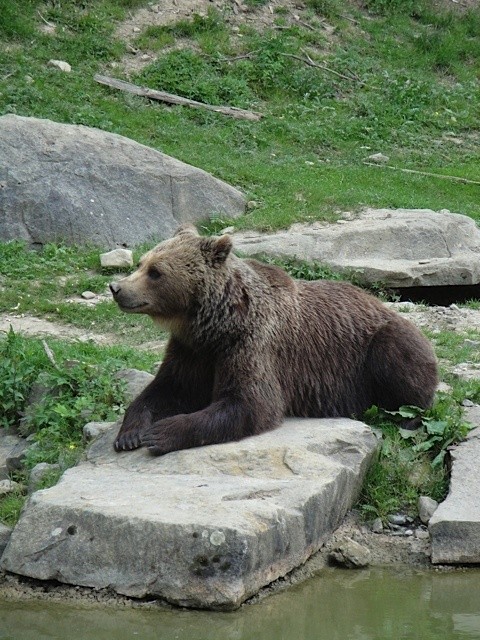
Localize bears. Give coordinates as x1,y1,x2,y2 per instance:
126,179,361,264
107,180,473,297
109,223,439,456
